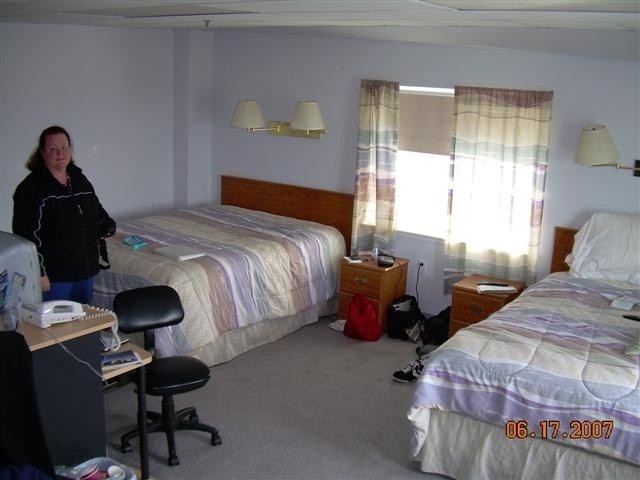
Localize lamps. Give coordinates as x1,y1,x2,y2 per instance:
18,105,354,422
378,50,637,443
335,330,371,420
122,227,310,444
231,100,328,139
576,124,640,177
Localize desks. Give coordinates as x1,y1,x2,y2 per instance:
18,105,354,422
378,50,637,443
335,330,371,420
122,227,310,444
0,306,153,480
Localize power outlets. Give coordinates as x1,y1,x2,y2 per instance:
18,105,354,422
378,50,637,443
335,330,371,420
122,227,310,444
416,259,426,274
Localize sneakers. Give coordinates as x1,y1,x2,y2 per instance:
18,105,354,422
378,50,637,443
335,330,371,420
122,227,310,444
393,353,429,383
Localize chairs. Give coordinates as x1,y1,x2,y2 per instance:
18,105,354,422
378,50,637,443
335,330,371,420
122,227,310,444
114,286,221,466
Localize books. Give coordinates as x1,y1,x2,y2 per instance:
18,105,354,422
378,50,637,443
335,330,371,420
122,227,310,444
478,284,518,295
152,244,206,262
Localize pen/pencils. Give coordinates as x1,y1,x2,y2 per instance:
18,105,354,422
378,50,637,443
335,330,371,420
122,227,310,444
477,283,508,286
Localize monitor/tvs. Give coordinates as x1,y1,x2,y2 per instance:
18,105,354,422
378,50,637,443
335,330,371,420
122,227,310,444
0,230,43,315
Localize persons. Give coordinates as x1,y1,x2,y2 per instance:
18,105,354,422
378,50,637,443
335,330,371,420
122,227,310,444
12,126,116,304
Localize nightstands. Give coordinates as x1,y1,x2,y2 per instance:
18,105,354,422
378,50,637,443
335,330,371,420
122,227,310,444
448,274,526,339
337,256,410,334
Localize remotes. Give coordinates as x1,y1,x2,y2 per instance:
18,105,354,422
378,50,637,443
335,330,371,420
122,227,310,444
477,280,508,286
623,315,640,321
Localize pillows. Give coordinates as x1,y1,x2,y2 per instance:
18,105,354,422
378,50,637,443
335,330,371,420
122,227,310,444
564,213,640,285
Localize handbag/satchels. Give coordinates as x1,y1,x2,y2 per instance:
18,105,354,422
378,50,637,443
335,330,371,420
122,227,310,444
389,294,427,343
425,305,450,345
344,292,381,341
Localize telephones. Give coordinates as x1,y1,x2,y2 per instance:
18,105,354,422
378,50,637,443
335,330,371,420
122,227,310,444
20,299,87,328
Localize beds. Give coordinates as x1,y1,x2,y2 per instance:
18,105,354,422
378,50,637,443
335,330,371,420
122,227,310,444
91,176,354,369
406,227,640,480
